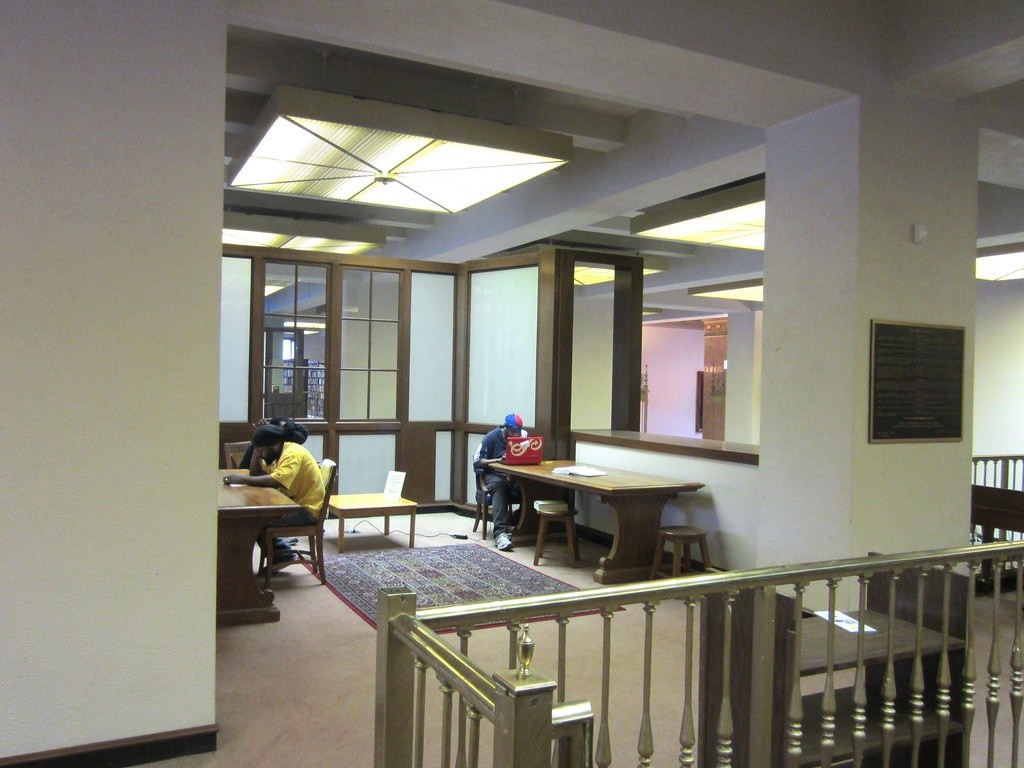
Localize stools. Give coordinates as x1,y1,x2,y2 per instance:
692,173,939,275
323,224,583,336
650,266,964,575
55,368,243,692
534,510,578,566
649,525,712,582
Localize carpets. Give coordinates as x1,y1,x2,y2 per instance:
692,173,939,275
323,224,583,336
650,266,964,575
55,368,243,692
292,531,626,636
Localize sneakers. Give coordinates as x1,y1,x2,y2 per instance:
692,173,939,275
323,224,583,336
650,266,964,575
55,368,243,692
494,533,512,550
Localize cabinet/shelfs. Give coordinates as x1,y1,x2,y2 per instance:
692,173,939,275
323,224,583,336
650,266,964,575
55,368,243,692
704,550,971,768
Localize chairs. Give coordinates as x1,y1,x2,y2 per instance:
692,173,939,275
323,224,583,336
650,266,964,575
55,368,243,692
224,441,252,470
257,458,337,588
473,474,523,540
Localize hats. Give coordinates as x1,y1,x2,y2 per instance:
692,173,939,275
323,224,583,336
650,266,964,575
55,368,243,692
253,424,282,447
505,414,523,431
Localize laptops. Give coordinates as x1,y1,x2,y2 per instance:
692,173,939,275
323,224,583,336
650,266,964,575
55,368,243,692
497,436,544,466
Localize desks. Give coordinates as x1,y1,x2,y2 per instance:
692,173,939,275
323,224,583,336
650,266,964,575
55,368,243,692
216,469,302,626
486,459,706,585
329,493,417,554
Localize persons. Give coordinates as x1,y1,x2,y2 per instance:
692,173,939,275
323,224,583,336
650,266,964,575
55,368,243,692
223,417,326,575
473,414,529,549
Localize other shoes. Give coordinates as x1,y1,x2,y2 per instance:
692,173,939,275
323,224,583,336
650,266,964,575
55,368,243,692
277,536,299,546
260,558,292,577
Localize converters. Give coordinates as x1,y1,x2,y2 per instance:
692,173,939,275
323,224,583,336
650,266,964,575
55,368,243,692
453,534,467,539
